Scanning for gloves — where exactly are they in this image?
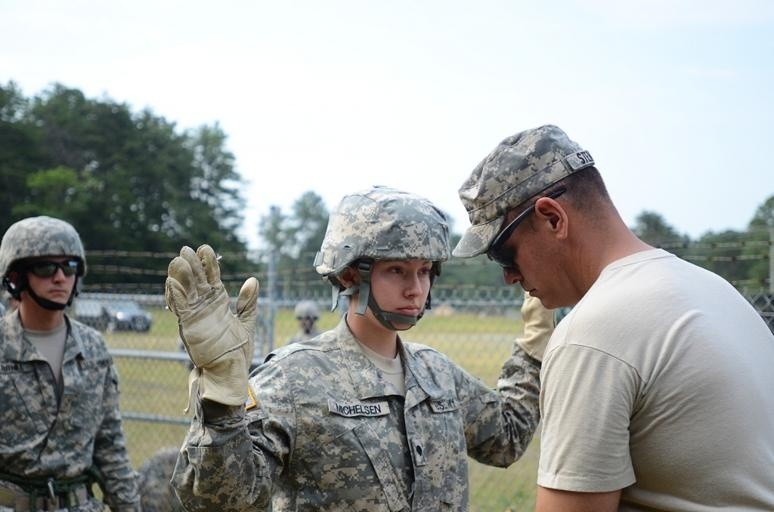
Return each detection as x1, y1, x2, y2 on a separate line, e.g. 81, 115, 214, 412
516, 291, 556, 362
165, 244, 260, 407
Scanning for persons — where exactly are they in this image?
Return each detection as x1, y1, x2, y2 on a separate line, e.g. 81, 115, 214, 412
132, 444, 186, 512
0, 215, 142, 512
164, 186, 557, 512
288, 299, 322, 343
453, 125, 774, 512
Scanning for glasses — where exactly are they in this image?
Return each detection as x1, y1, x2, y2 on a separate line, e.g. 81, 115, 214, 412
29, 260, 81, 278
486, 188, 567, 268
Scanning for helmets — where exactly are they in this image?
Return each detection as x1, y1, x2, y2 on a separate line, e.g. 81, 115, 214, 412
313, 184, 449, 276
295, 300, 320, 319
0, 216, 87, 290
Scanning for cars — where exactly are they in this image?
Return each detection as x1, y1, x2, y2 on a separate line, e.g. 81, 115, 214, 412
70, 297, 152, 332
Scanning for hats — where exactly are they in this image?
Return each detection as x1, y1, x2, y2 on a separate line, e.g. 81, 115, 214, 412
452, 124, 596, 258
